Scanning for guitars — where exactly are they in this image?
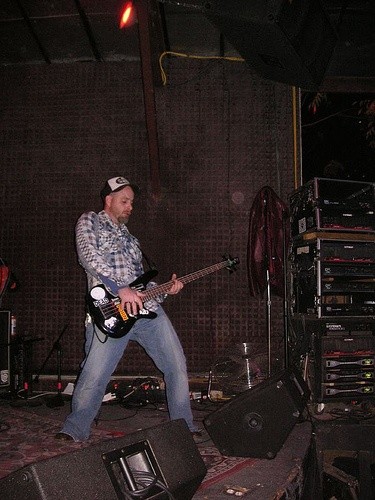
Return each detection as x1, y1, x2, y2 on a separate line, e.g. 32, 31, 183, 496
85, 254, 240, 338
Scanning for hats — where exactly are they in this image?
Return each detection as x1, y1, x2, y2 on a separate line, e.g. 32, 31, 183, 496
102, 176, 140, 199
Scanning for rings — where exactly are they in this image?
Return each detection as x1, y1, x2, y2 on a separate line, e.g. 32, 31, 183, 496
125, 302, 131, 304
132, 301, 136, 303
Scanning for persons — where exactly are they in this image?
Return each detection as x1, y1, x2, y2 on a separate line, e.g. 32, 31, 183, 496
55, 176, 204, 444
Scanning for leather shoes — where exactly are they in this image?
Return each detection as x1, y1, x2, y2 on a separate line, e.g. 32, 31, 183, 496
191, 432, 203, 438
54, 432, 74, 443
